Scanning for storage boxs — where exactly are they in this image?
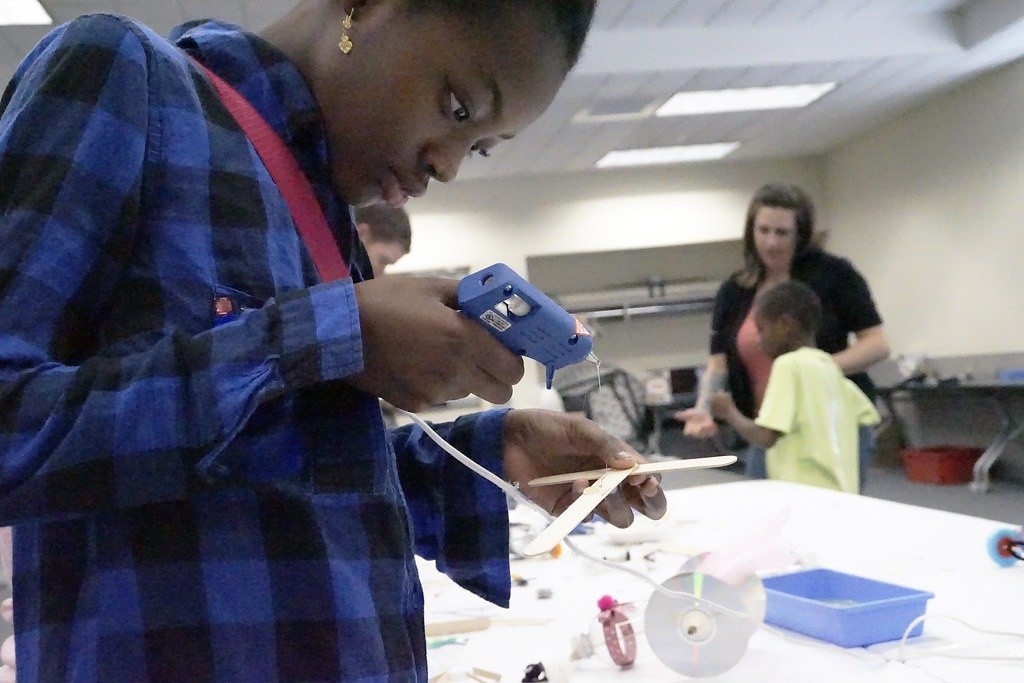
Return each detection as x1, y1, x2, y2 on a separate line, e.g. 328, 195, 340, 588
760, 567, 935, 646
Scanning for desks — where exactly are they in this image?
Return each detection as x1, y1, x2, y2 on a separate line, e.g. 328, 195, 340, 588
368, 479, 1024, 683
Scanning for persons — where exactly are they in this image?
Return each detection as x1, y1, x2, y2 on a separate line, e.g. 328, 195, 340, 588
0, 0, 667, 683
351, 201, 410, 277
675, 180, 891, 495
